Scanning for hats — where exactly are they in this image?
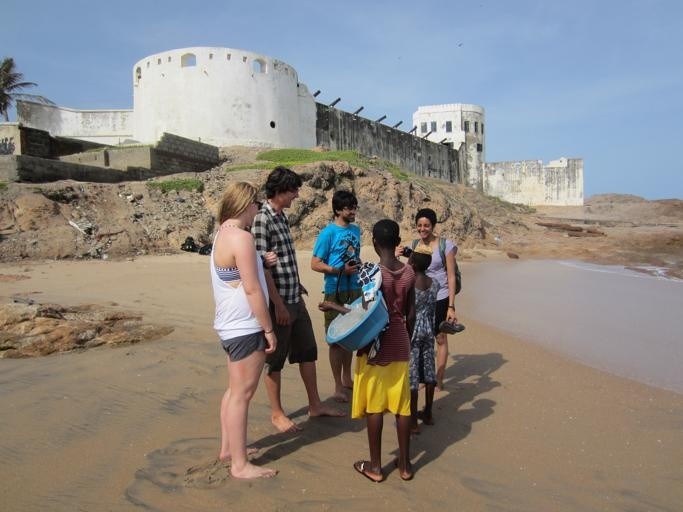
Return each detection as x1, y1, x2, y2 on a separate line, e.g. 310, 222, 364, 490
414, 245, 433, 256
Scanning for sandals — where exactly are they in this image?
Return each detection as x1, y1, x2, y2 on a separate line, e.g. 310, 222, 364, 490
439, 321, 465, 334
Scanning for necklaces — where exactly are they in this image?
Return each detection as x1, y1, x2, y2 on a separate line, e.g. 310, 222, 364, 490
217, 224, 244, 229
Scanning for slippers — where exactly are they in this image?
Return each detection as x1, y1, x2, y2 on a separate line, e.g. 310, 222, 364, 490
354, 461, 383, 482
394, 457, 413, 480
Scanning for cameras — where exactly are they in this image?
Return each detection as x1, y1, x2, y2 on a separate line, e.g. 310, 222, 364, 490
400, 246, 412, 257
348, 258, 357, 268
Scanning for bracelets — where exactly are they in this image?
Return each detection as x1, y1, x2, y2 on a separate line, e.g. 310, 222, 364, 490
447, 305, 454, 310
264, 327, 274, 336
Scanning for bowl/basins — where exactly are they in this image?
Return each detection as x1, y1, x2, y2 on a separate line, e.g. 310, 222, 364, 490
325, 289, 391, 352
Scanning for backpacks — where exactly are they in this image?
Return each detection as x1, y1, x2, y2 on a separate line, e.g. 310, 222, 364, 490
412, 238, 461, 294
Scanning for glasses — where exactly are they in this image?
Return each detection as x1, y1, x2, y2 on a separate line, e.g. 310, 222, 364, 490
344, 205, 360, 211
255, 201, 263, 210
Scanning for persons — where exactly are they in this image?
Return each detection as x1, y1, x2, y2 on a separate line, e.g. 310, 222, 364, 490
395, 207, 461, 393
209, 181, 280, 481
310, 189, 366, 405
247, 164, 348, 437
401, 244, 441, 430
316, 217, 417, 484
